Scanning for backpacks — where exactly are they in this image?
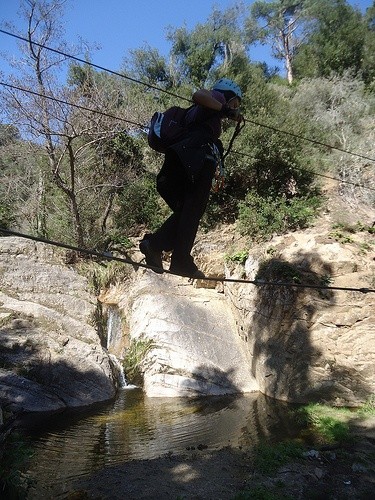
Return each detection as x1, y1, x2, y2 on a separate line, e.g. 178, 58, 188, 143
147, 106, 184, 153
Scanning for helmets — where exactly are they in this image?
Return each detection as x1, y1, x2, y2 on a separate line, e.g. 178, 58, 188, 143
212, 77, 242, 101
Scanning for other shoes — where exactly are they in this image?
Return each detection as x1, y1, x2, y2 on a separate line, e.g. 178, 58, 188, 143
169, 258, 205, 278
138, 233, 166, 274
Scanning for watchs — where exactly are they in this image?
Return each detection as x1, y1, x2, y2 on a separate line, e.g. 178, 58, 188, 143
226, 108, 232, 118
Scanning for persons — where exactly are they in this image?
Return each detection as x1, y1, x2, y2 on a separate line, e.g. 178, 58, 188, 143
139, 77, 246, 278
